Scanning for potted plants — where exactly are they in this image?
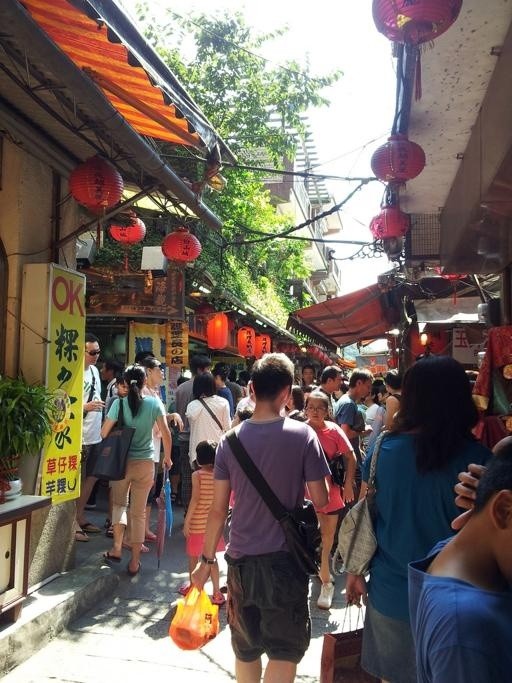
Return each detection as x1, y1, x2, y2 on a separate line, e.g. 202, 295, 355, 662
0, 367, 59, 501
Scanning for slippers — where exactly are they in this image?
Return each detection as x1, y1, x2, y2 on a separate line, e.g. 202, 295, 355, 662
75, 522, 103, 542
128, 559, 141, 577
103, 551, 121, 563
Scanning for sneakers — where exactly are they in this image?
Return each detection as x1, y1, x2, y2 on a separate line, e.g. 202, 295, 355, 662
179, 585, 190, 597
211, 592, 225, 605
318, 583, 334, 609
171, 493, 177, 503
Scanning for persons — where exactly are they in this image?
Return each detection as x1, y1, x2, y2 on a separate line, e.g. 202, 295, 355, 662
73, 333, 109, 543
98, 351, 405, 612
342, 353, 495, 683
406, 434, 512, 683
189, 351, 333, 683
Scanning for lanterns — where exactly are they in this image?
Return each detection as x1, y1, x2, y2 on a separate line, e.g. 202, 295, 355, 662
367, 205, 412, 241
206, 309, 229, 350
368, 133, 428, 193
236, 324, 256, 357
109, 211, 148, 271
278, 342, 334, 367
67, 154, 128, 250
437, 266, 468, 306
254, 333, 271, 360
160, 224, 202, 294
194, 301, 216, 326
372, 0, 463, 102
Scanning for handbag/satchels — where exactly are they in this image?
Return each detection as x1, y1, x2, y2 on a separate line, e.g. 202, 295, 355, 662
337, 496, 377, 578
279, 499, 323, 575
320, 628, 381, 683
86, 423, 136, 481
329, 454, 361, 489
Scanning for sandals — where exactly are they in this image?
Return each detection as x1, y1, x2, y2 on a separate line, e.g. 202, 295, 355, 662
104, 518, 157, 553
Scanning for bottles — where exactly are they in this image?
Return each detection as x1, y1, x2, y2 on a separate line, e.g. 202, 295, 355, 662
317, 582, 336, 610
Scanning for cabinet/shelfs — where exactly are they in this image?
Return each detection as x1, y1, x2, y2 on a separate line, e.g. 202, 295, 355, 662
0, 494, 52, 622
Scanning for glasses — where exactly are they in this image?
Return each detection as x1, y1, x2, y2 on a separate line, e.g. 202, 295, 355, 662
307, 405, 326, 412
85, 350, 101, 357
153, 364, 166, 371
